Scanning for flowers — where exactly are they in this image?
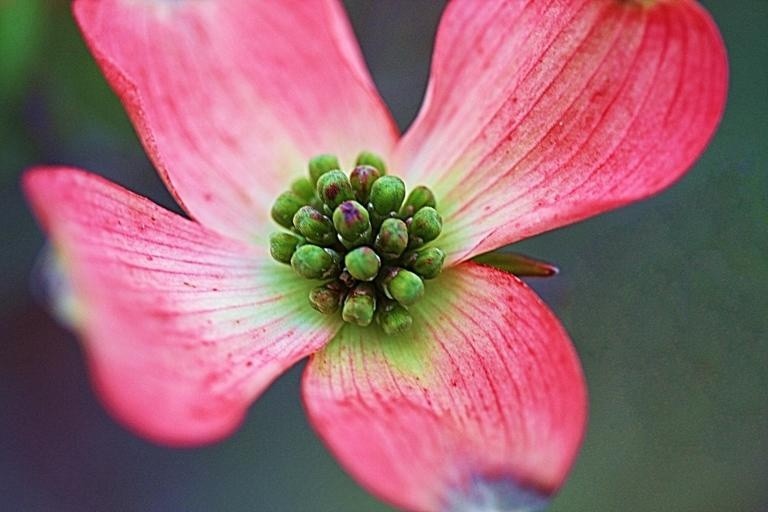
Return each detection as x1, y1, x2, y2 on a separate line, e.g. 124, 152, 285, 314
20, 0, 731, 512
